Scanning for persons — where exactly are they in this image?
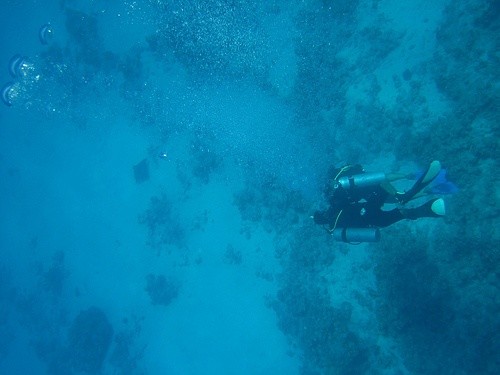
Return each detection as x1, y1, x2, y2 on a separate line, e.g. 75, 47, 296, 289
309, 158, 449, 230
